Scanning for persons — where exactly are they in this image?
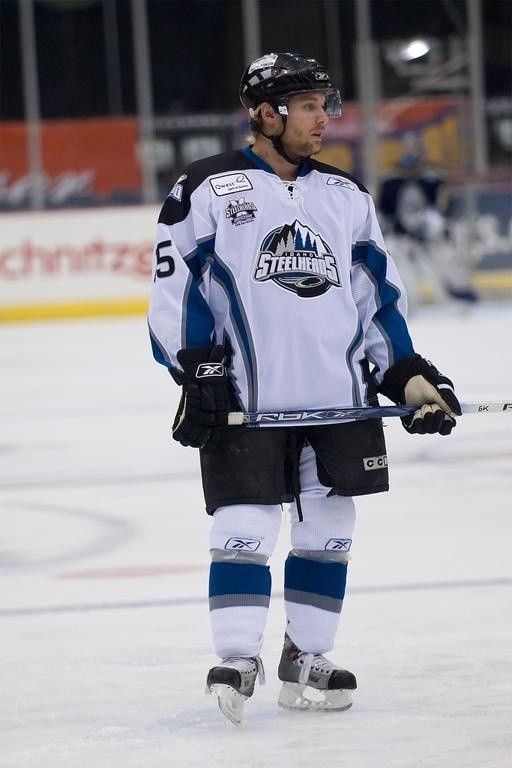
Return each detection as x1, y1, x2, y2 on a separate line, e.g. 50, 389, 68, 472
147, 49, 463, 697
376, 146, 478, 304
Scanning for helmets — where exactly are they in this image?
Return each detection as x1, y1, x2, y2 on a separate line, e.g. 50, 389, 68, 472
239, 52, 334, 115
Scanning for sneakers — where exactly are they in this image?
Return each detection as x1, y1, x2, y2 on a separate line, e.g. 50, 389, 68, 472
280, 632, 358, 691
206, 654, 266, 699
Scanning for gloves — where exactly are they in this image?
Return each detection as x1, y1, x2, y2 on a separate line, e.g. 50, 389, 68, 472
172, 344, 246, 454
376, 354, 463, 435
